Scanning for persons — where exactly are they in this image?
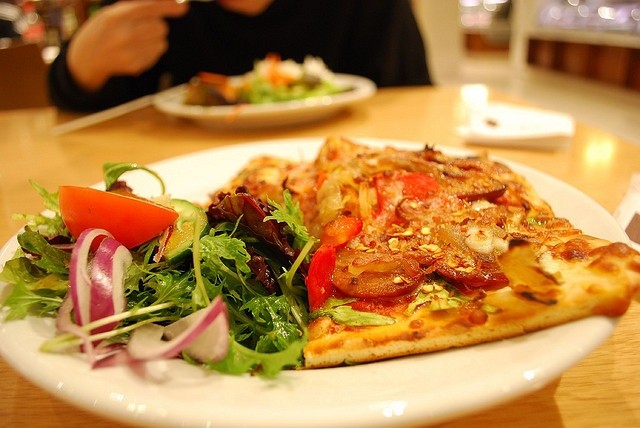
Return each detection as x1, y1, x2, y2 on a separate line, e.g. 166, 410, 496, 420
47, 0, 432, 114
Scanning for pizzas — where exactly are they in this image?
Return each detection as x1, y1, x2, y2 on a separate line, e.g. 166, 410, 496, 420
215, 150, 316, 235
300, 131, 637, 371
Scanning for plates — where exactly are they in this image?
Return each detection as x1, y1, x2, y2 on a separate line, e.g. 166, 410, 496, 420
0, 133, 640, 427
150, 68, 379, 132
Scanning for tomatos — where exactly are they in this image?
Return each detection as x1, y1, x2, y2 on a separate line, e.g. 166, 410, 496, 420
56, 184, 180, 258
